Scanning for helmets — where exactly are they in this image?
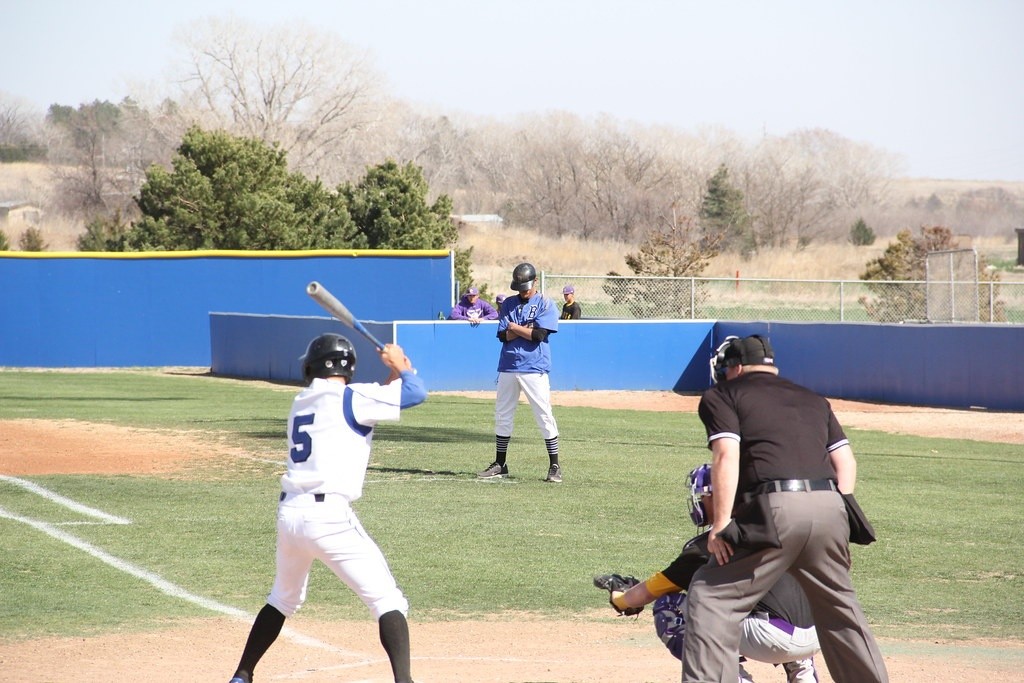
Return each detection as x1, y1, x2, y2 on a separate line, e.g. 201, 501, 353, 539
510, 263, 536, 290
299, 334, 357, 383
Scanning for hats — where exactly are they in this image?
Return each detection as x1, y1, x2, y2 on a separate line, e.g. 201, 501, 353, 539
496, 295, 506, 303
717, 334, 775, 367
561, 286, 574, 294
466, 288, 477, 296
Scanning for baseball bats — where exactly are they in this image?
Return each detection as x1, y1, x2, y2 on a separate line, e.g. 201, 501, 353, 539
305, 279, 418, 376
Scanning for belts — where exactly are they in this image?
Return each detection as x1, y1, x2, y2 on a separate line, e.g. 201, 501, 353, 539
769, 618, 795, 635
767, 479, 839, 492
280, 492, 325, 502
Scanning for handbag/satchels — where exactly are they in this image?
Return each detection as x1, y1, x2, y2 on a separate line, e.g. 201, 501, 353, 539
716, 492, 782, 549
842, 494, 876, 544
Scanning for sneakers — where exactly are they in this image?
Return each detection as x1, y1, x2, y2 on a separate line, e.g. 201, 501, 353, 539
478, 462, 508, 479
547, 464, 562, 483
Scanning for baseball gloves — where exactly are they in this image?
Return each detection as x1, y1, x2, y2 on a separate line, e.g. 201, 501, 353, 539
593, 573, 644, 615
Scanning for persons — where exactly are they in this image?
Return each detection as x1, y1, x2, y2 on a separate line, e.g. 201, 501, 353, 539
561, 286, 581, 319
496, 294, 506, 310
681, 331, 890, 683
228, 334, 428, 683
479, 264, 562, 482
451, 287, 498, 323
611, 464, 821, 683
